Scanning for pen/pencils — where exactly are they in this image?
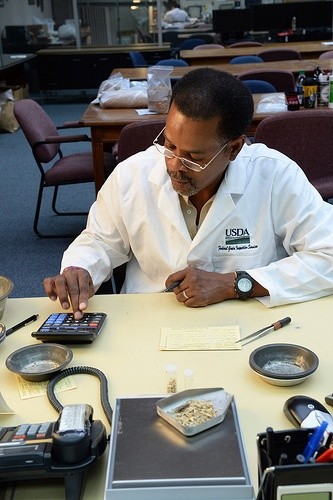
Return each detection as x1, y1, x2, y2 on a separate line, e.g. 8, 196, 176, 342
266, 421, 333, 465
6, 314, 39, 336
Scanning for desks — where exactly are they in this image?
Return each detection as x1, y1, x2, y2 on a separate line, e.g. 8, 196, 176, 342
110, 59, 333, 89
80, 92, 333, 201
177, 40, 333, 65
0, 53, 38, 85
0, 290, 333, 500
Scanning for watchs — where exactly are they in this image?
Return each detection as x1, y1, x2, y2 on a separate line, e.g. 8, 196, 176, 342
234, 271, 254, 300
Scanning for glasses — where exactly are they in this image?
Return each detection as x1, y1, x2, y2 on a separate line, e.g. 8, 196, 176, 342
152, 126, 227, 172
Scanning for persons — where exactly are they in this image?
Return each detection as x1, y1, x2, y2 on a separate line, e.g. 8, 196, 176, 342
42, 68, 333, 319
162, 2, 192, 23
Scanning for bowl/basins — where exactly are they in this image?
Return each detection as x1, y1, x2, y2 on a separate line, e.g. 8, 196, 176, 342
248, 343, 319, 386
5, 343, 74, 382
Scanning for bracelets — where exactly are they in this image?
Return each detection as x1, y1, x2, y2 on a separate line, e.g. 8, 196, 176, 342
63, 267, 81, 271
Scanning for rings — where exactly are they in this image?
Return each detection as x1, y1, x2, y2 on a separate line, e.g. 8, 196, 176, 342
183, 290, 189, 299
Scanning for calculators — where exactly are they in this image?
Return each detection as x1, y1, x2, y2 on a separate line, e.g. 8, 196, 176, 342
32, 313, 107, 344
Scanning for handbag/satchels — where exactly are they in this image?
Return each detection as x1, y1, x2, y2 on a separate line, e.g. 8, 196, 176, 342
0, 100, 20, 133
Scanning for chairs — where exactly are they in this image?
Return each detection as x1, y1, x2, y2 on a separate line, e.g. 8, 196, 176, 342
14, 35, 333, 237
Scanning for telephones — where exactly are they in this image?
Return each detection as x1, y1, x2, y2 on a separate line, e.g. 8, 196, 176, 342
0, 404, 107, 500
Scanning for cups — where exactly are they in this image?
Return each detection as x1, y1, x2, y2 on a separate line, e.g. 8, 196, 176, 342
284, 91, 301, 111
302, 78, 318, 109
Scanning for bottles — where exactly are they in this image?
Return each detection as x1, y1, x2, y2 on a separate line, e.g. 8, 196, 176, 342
314, 66, 333, 108
295, 69, 306, 104
292, 17, 296, 30
